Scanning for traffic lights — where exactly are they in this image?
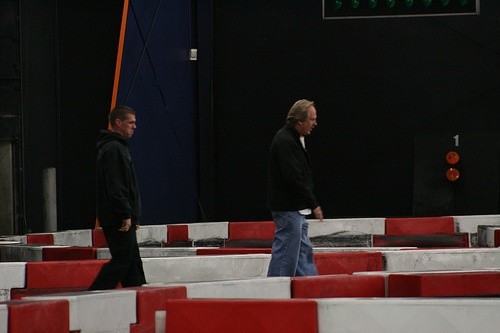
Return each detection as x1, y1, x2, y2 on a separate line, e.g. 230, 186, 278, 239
429, 145, 467, 183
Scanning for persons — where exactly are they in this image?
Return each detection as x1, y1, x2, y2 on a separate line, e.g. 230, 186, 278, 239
266, 99, 324, 278
88, 103, 147, 292
